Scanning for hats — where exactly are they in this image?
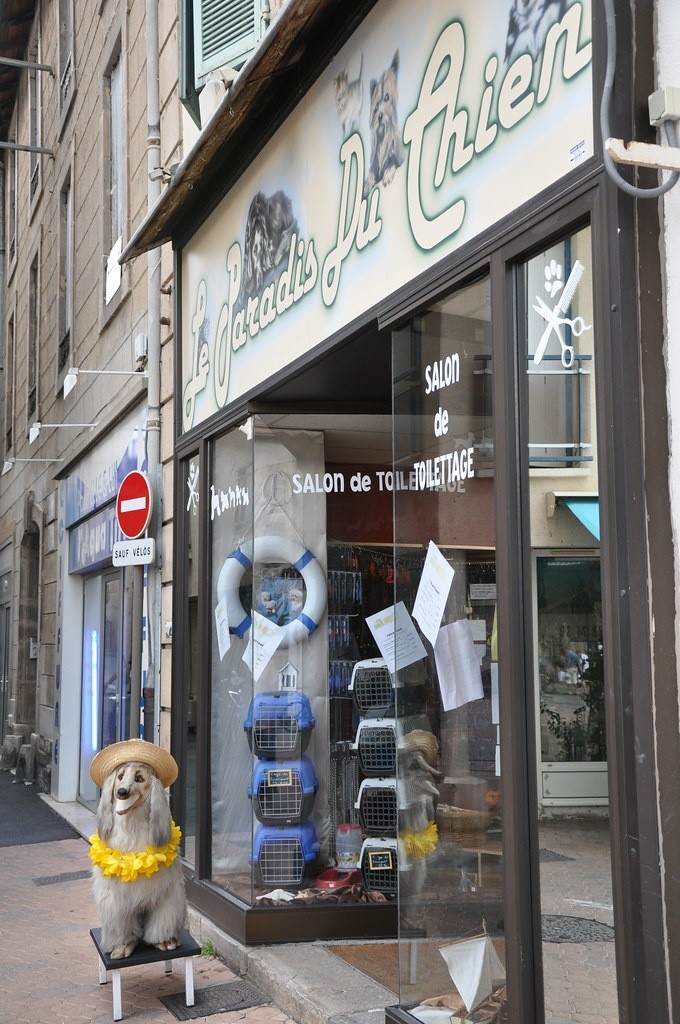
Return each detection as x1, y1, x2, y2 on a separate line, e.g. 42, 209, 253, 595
90, 739, 178, 788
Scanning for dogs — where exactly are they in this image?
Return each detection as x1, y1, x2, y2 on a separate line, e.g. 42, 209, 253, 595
87, 760, 189, 960
398, 751, 446, 930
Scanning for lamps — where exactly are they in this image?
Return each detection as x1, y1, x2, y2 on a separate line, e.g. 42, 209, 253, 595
63, 367, 147, 401
1, 457, 63, 478
29, 422, 96, 447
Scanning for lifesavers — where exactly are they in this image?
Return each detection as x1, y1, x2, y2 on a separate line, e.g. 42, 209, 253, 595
215, 534, 329, 651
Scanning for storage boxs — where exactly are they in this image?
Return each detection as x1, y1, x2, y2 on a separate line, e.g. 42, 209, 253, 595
243, 690, 322, 889
348, 659, 434, 898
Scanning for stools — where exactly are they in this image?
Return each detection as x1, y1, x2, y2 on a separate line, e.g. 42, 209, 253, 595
90, 925, 201, 1022
